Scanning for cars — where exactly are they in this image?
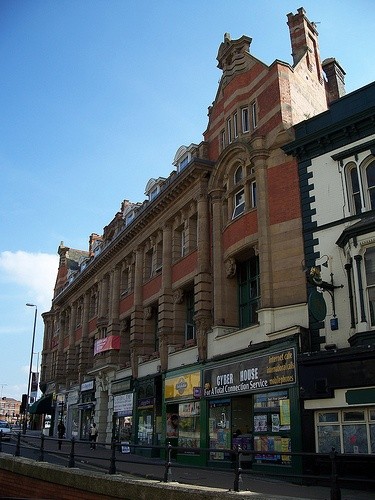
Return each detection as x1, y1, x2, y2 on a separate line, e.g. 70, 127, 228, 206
0, 421, 13, 440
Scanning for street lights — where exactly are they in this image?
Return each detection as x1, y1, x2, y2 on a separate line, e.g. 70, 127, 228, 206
23, 303, 37, 436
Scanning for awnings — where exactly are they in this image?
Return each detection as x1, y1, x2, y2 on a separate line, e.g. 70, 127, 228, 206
29, 392, 55, 415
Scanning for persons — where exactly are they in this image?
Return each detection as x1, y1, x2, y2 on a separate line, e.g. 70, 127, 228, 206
58, 420, 66, 444
89, 422, 98, 450
